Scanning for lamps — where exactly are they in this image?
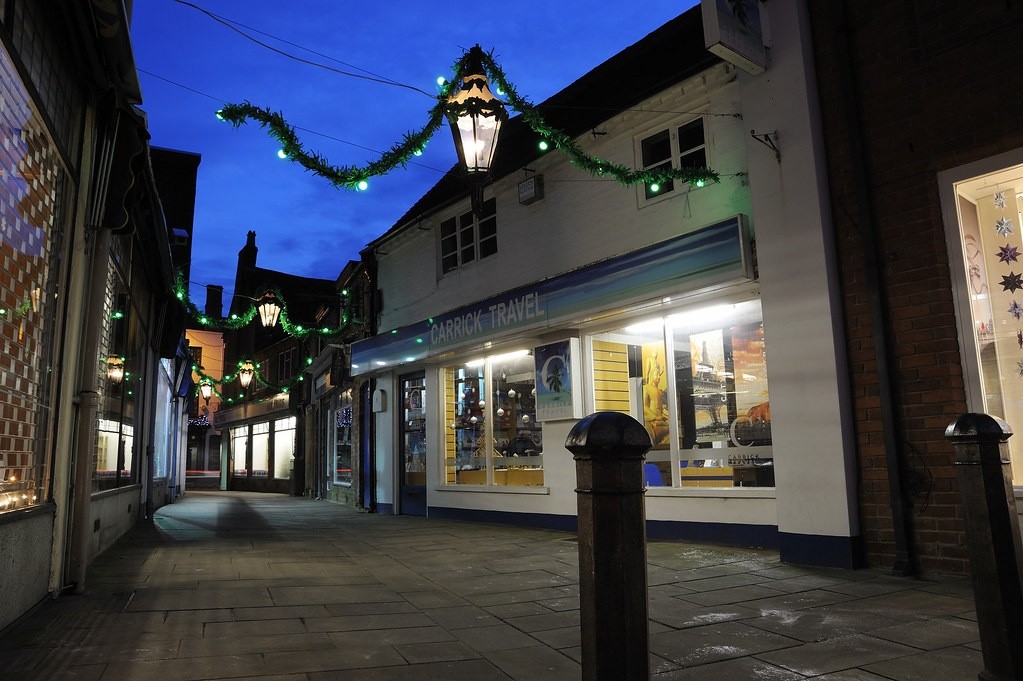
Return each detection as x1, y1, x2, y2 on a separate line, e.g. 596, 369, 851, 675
200, 381, 212, 405
238, 358, 255, 389
255, 284, 283, 336
443, 43, 498, 218
